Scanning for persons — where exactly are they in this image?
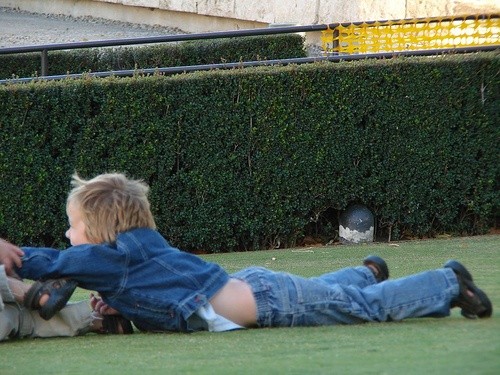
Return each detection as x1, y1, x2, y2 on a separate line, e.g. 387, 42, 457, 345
0, 236, 135, 341
0, 172, 493, 334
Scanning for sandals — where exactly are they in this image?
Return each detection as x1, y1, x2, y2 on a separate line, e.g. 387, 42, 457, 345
363, 254, 390, 282
444, 260, 492, 319
100, 314, 133, 334
32, 278, 79, 320
